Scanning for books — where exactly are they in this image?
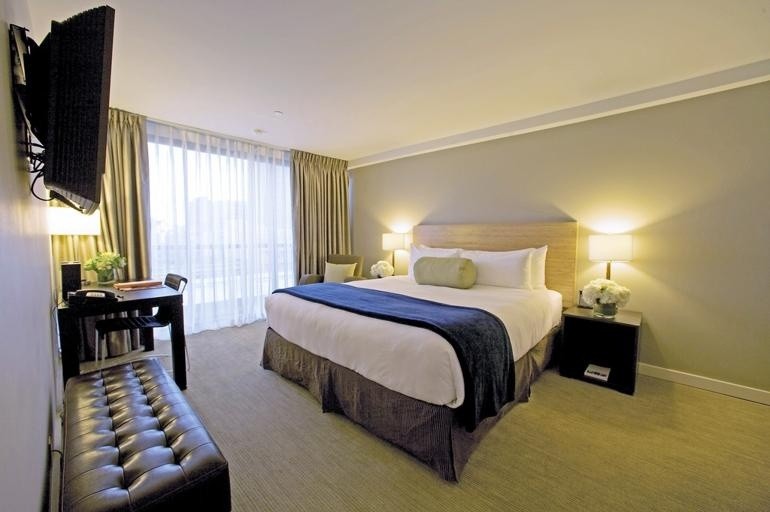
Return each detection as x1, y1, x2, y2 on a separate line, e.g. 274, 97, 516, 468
114, 280, 164, 292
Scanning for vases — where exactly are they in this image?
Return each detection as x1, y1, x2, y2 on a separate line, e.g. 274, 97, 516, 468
97, 270, 115, 285
593, 302, 616, 318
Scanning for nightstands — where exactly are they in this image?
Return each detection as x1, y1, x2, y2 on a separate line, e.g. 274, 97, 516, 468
560, 305, 643, 396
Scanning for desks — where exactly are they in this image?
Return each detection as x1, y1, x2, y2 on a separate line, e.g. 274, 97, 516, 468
58, 280, 186, 390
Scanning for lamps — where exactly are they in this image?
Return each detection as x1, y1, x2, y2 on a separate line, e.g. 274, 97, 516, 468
382, 233, 406, 268
48, 207, 101, 262
588, 235, 633, 280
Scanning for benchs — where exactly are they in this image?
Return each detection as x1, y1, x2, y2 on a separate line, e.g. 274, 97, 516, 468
62, 357, 231, 512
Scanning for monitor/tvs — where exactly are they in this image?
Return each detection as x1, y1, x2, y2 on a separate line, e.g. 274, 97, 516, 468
21, 5, 116, 217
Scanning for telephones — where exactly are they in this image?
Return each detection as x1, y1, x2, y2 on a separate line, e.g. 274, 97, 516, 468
67, 289, 117, 305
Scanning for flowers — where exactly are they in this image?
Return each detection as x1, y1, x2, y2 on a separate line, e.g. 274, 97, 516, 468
83, 251, 126, 270
583, 278, 630, 309
370, 261, 395, 278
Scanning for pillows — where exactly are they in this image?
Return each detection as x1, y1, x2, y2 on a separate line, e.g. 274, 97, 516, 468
324, 260, 357, 284
420, 245, 462, 255
530, 245, 548, 291
459, 248, 535, 288
408, 244, 458, 286
414, 257, 477, 289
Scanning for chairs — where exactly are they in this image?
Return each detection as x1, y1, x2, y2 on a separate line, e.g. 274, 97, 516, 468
95, 274, 188, 371
298, 254, 367, 284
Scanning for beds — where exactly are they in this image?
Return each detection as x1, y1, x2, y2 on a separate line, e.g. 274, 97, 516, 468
265, 223, 579, 470
